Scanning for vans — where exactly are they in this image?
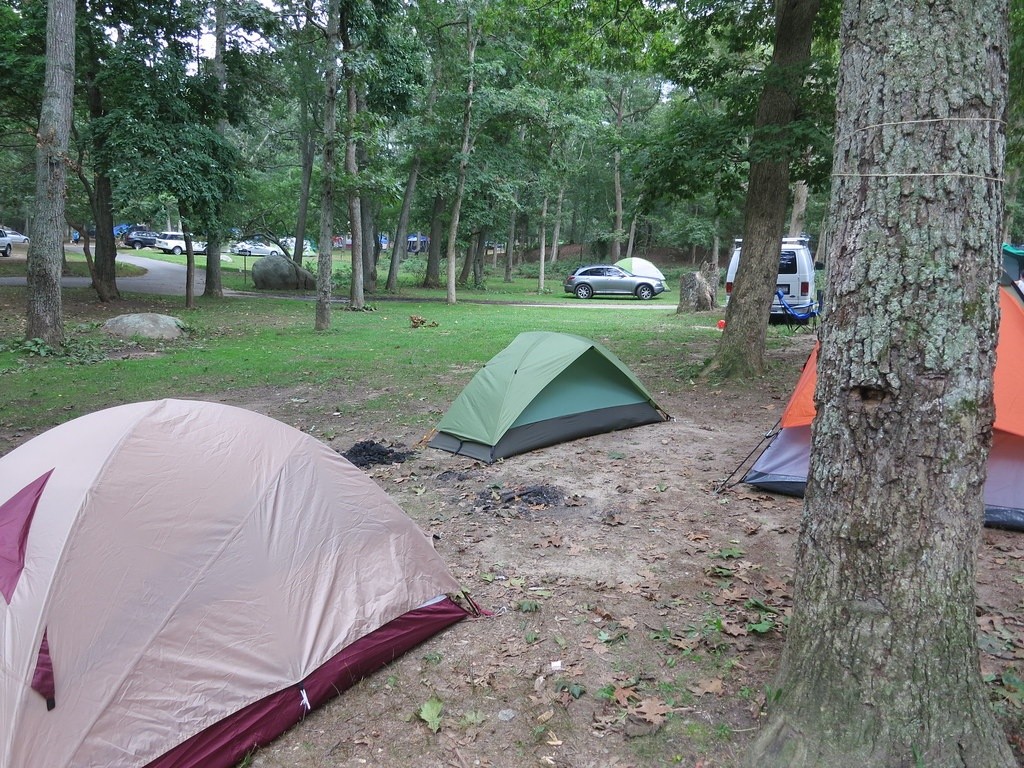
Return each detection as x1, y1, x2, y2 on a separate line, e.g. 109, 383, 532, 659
726, 238, 812, 317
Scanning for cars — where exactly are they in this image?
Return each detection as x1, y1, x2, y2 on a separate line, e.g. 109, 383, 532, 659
5, 231, 30, 243
231, 242, 279, 256
0, 229, 12, 256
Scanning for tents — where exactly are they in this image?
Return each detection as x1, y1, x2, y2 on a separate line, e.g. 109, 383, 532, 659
425, 328, 672, 465
613, 257, 671, 292
0, 396, 481, 768
742, 255, 1024, 533
373, 232, 432, 253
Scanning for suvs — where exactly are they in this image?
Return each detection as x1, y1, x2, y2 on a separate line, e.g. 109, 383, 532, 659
124, 231, 160, 248
565, 265, 663, 300
155, 232, 207, 255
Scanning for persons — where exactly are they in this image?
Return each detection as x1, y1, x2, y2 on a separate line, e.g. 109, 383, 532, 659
73, 230, 81, 246
1012, 270, 1024, 294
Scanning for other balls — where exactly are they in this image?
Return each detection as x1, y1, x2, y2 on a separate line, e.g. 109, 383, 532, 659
717, 319, 725, 329
